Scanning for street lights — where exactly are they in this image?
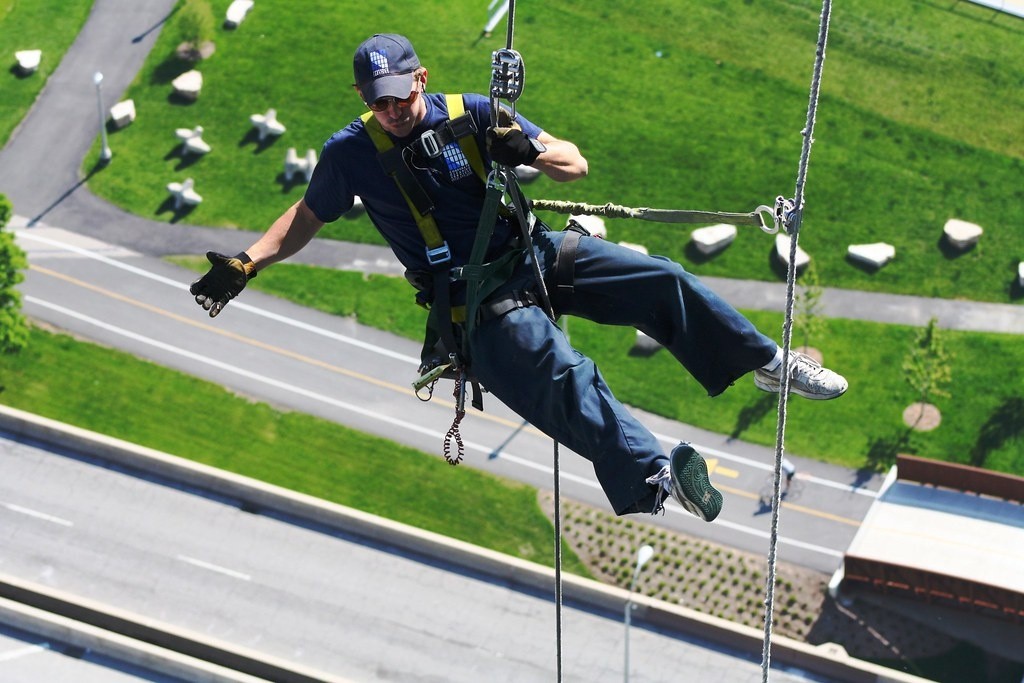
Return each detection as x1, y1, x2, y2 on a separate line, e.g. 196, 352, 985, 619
92, 71, 113, 163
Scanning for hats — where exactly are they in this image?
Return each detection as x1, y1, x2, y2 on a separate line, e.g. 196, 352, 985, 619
353, 33, 420, 106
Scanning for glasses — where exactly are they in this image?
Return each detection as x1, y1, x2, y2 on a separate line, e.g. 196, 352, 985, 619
361, 74, 421, 112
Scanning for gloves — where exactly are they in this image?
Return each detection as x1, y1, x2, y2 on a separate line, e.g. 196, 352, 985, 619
486, 106, 546, 167
190, 250, 258, 318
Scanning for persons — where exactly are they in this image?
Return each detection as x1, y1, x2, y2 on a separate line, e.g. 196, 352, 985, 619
189, 33, 849, 523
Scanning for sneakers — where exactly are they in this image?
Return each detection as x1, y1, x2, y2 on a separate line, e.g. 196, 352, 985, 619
754, 350, 848, 400
646, 443, 723, 523
